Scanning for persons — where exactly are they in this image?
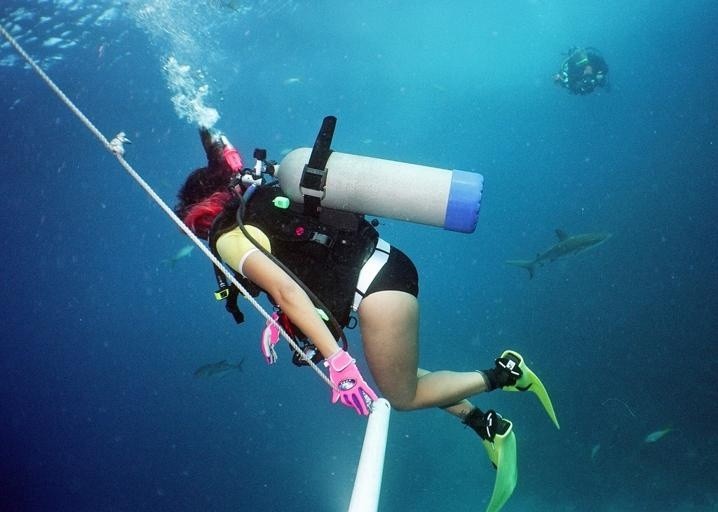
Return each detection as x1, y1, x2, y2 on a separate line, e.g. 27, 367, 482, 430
552, 45, 609, 95
172, 167, 562, 512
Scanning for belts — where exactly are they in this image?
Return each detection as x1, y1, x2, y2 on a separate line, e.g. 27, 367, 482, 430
352, 236, 391, 313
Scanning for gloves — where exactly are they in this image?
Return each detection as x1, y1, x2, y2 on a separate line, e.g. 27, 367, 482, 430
260, 314, 282, 364
324, 348, 378, 417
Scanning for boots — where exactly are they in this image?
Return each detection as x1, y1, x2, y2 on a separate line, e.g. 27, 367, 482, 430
474, 348, 562, 430
463, 408, 518, 512
